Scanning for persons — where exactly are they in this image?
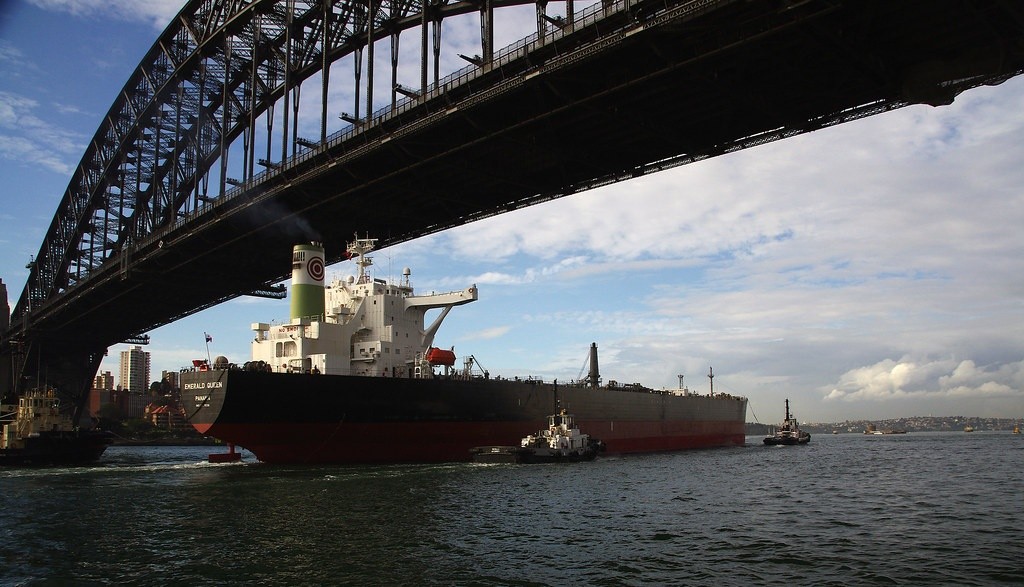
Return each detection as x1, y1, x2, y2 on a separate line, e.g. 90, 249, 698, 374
286, 365, 293, 373
312, 365, 320, 373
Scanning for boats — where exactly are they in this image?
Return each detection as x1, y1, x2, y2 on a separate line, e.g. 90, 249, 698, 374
179, 231, 749, 466
763, 398, 811, 445
0, 387, 115, 469
964, 425, 973, 432
520, 378, 602, 463
832, 431, 837, 434
1013, 427, 1021, 434
468, 445, 536, 464
863, 424, 908, 434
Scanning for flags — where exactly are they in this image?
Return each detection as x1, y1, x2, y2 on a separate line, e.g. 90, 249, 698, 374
205, 334, 212, 343
